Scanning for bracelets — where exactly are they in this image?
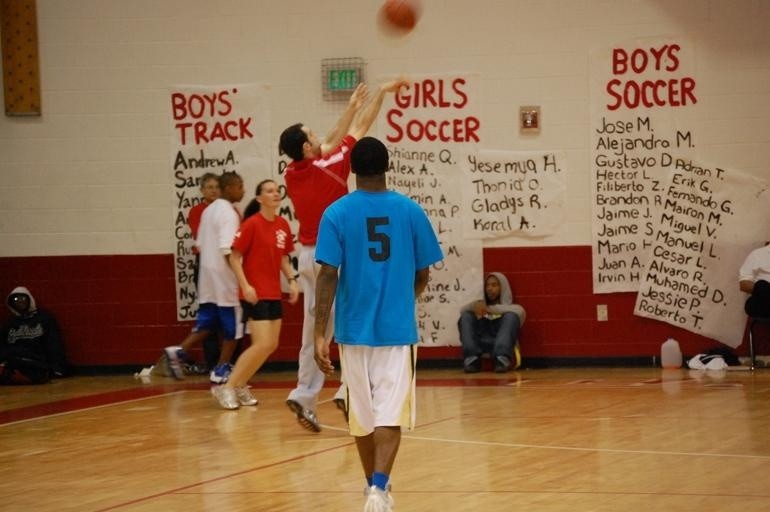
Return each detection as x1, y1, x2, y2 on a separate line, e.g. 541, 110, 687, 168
287, 278, 295, 283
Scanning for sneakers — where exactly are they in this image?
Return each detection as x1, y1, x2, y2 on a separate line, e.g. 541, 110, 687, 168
165, 346, 259, 410
495, 363, 505, 373
364, 485, 392, 512
334, 388, 348, 420
286, 398, 321, 432
464, 362, 480, 373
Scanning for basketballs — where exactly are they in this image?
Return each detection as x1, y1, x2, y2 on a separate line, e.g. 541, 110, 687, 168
376, 1, 422, 36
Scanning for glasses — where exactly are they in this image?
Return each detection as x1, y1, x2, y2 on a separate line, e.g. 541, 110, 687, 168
13, 297, 27, 302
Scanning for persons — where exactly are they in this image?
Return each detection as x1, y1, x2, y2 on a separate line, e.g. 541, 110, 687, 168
161, 173, 253, 382
0, 280, 64, 386
459, 271, 526, 374
189, 174, 246, 366
211, 180, 300, 410
738, 241, 770, 319
279, 79, 410, 431
310, 138, 443, 511
